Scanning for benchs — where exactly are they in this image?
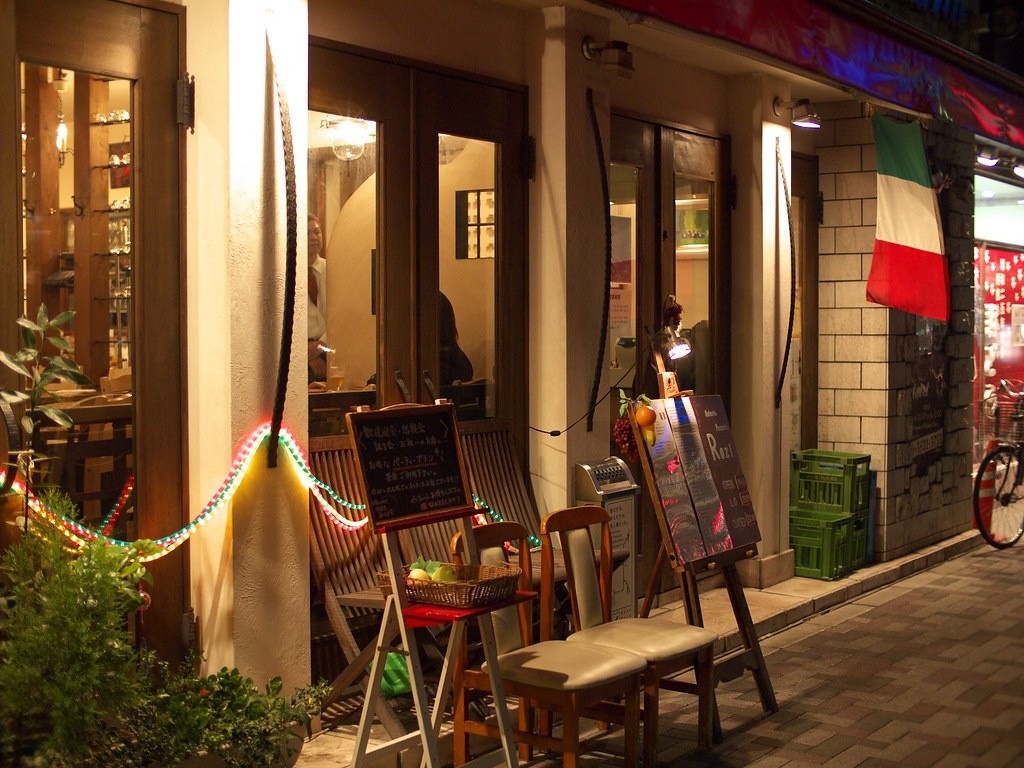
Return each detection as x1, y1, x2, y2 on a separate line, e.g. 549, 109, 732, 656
309, 419, 631, 748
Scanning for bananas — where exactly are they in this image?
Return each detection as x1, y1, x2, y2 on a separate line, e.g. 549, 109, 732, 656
640, 400, 656, 446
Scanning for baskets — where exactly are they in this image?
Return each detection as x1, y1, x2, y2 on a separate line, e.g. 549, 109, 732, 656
979, 400, 1024, 444
379, 562, 523, 608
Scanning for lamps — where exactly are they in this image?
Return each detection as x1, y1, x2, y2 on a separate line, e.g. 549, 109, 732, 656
332, 116, 365, 161
1013, 159, 1024, 180
772, 95, 826, 133
660, 316, 699, 364
50, 113, 71, 168
976, 145, 1001, 168
584, 34, 639, 79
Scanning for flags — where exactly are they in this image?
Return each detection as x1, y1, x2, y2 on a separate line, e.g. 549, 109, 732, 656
867, 113, 950, 322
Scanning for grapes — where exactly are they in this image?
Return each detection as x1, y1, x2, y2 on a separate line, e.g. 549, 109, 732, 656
613, 417, 637, 455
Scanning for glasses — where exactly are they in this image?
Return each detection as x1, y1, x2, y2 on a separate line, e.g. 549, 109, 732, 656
96, 108, 134, 299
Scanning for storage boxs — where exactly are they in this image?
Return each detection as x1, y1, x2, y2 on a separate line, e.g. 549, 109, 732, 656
790, 448, 881, 581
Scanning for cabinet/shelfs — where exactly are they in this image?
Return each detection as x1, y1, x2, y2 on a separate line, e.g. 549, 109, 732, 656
87, 118, 130, 346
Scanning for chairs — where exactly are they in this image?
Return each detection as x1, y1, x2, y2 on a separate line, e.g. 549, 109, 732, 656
536, 506, 721, 768
445, 521, 649, 768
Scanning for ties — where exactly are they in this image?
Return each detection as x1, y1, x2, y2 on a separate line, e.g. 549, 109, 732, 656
308, 266, 318, 305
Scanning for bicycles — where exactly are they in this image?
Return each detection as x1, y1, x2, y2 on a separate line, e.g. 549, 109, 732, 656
972, 378, 1024, 550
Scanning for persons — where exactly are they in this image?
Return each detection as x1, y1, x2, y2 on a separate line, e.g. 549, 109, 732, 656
307, 214, 327, 384
438, 291, 473, 385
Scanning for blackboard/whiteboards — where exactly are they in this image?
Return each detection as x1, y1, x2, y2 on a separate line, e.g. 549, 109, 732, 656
346, 403, 474, 532
627, 395, 762, 572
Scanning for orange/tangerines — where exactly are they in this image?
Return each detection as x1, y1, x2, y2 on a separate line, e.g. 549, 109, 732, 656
407, 569, 430, 587
635, 405, 656, 427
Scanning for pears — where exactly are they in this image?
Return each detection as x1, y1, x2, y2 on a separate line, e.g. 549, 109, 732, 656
431, 566, 456, 589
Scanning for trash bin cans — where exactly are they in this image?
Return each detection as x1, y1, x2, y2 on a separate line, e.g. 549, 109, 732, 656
574, 456, 641, 618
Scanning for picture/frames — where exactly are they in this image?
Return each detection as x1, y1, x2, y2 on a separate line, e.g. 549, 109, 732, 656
675, 200, 709, 259
